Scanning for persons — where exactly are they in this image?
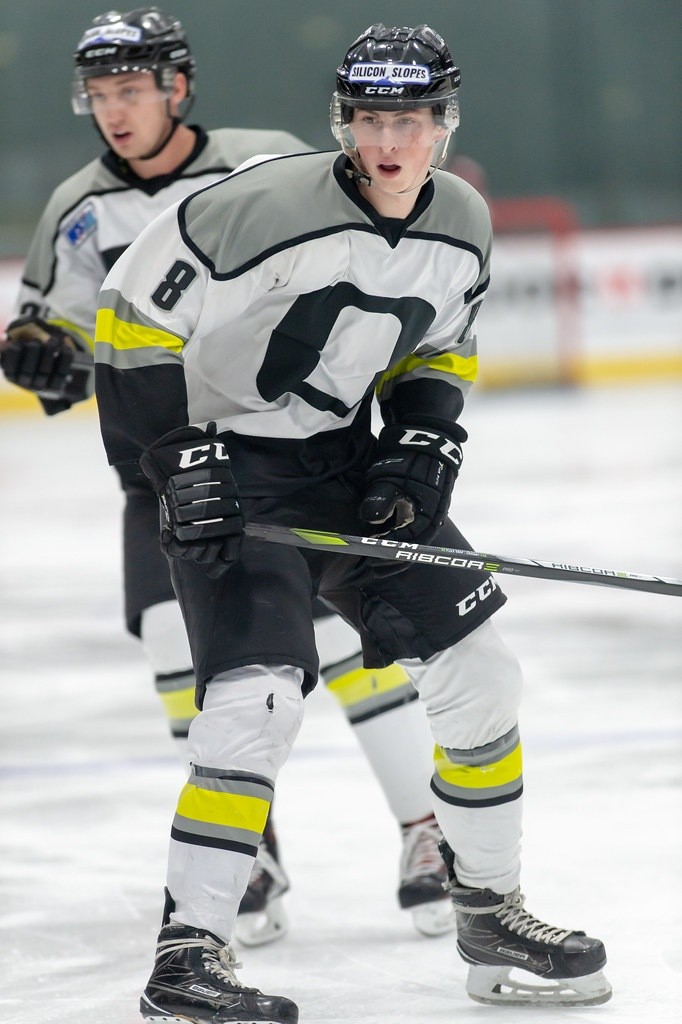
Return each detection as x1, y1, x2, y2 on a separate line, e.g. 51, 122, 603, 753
0, 9, 456, 947
96, 22, 614, 1023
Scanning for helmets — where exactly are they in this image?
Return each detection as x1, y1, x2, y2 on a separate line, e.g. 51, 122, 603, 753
337, 22, 461, 130
74, 6, 195, 98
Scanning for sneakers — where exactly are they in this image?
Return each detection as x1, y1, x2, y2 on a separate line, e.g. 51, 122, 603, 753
398, 811, 459, 938
235, 815, 290, 946
437, 837, 612, 1009
140, 886, 299, 1024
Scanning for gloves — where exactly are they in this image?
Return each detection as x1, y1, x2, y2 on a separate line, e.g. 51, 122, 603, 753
0, 317, 89, 401
360, 413, 468, 578
139, 421, 246, 579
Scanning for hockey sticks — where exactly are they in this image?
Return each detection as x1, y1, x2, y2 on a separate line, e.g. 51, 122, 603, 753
0, 340, 94, 371
242, 521, 682, 599
